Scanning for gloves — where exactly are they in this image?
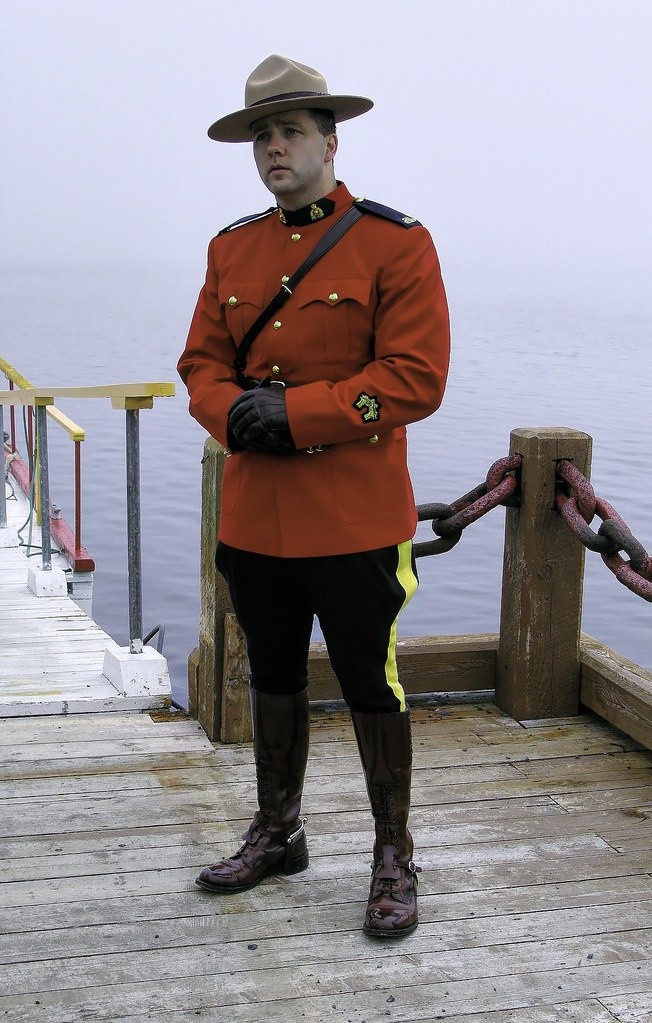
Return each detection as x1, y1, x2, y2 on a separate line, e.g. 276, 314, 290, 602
229, 387, 296, 456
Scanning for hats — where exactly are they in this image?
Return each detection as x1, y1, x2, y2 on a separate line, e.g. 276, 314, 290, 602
208, 54, 374, 144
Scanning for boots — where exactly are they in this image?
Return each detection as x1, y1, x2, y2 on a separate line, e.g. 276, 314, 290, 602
196, 685, 309, 894
350, 702, 423, 937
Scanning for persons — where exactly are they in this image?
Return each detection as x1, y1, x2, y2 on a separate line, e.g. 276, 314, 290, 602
176, 54, 451, 935
